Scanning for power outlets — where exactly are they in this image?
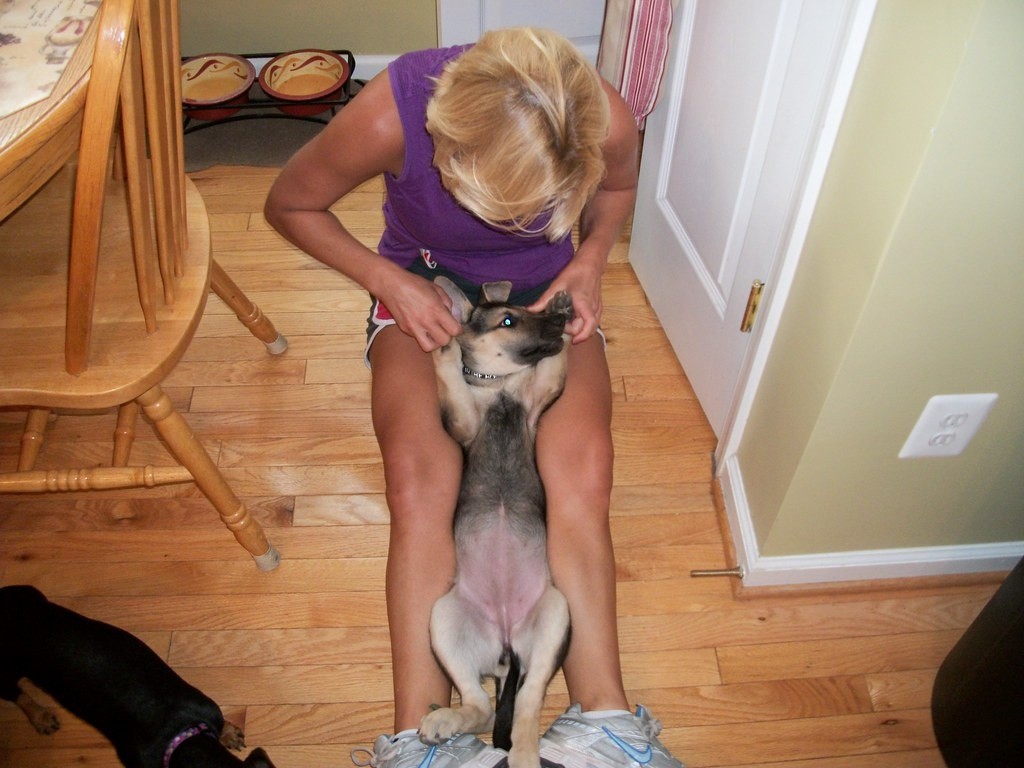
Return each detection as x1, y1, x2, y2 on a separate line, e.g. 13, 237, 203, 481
898, 392, 999, 459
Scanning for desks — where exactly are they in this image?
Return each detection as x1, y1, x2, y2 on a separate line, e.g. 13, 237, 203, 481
0, 0, 115, 224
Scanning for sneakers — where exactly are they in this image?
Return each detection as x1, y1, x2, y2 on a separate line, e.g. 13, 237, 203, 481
350, 729, 508, 768
539, 703, 689, 768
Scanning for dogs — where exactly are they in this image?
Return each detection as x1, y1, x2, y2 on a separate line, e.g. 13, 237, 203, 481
416, 275, 573, 768
0, 584, 276, 768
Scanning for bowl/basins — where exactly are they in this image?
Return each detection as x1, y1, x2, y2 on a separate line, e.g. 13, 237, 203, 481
258, 48, 350, 117
178, 52, 256, 122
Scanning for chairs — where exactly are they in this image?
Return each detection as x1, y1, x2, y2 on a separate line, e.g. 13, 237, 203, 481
0, 0, 289, 575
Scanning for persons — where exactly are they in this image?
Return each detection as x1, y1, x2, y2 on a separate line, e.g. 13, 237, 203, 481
262, 27, 688, 768
